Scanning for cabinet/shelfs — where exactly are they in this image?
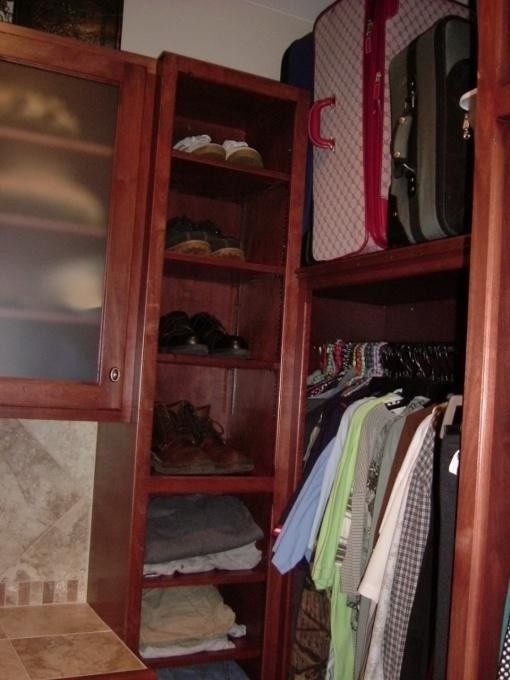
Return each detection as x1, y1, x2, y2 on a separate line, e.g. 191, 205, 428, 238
280, 1, 510, 680
0, 23, 161, 430
88, 51, 303, 662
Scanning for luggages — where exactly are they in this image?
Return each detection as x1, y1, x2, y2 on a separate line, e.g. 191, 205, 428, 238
171, 131, 227, 161
387, 11, 475, 245
306, 0, 471, 263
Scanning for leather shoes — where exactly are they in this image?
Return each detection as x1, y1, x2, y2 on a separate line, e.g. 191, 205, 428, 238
189, 310, 252, 359
156, 307, 213, 359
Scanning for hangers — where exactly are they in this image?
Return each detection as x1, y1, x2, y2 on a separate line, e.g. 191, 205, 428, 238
305, 336, 463, 404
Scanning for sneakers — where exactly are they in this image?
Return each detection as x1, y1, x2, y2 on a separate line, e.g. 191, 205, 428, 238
199, 217, 246, 262
164, 211, 213, 258
147, 400, 224, 476
190, 402, 257, 475
221, 138, 264, 168
